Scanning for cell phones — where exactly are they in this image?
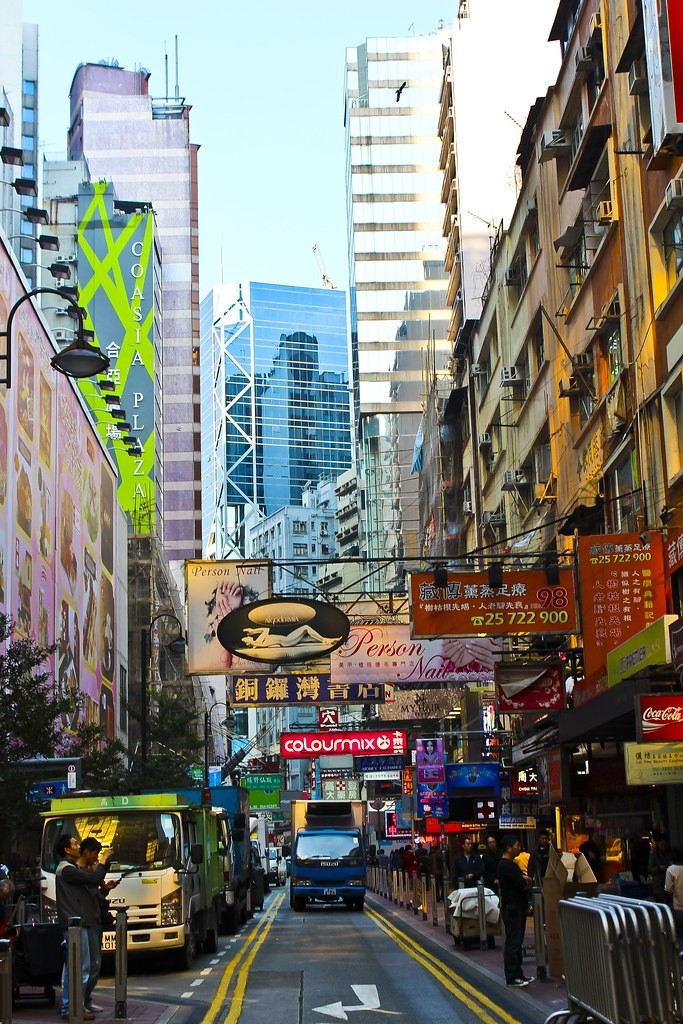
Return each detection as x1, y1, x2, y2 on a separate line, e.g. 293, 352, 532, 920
112, 878, 122, 887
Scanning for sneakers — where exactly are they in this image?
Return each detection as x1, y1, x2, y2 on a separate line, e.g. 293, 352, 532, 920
507, 976, 535, 987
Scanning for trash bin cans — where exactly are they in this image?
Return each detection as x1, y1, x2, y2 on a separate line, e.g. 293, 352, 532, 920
618, 879, 653, 901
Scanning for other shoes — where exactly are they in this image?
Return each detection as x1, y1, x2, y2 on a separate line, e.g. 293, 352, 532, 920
82, 1012, 95, 1020
90, 1004, 104, 1012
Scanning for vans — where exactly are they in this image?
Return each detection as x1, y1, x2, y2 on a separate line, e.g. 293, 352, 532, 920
269, 847, 287, 886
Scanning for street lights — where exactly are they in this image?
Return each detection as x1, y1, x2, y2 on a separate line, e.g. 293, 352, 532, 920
141, 614, 186, 769
204, 702, 237, 787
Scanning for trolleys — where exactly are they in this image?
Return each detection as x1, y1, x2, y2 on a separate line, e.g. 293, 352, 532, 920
450, 895, 501, 949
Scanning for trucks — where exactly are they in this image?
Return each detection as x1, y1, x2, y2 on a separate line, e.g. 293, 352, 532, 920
282, 800, 377, 911
142, 786, 250, 934
250, 816, 269, 892
23, 792, 246, 971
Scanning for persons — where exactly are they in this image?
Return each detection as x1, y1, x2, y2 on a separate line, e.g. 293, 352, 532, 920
366, 829, 549, 928
438, 638, 504, 672
420, 740, 441, 779
210, 581, 245, 667
241, 625, 342, 647
497, 835, 535, 987
648, 836, 683, 958
26, 833, 120, 1020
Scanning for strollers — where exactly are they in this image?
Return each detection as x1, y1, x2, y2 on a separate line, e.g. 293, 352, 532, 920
2, 922, 69, 1004
